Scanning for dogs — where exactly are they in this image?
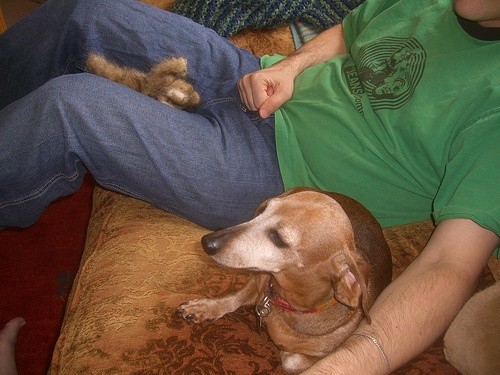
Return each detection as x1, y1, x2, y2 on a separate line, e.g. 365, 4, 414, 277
175, 186, 393, 375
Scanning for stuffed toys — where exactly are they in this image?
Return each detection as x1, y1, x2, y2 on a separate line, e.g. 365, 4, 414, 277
84, 49, 201, 109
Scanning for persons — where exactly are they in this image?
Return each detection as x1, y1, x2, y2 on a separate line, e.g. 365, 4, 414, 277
0, 0, 500, 375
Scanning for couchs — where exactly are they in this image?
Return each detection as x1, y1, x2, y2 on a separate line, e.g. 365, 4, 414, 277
47, 0, 500, 375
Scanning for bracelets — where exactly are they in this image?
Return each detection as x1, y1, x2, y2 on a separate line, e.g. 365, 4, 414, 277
348, 332, 393, 374
